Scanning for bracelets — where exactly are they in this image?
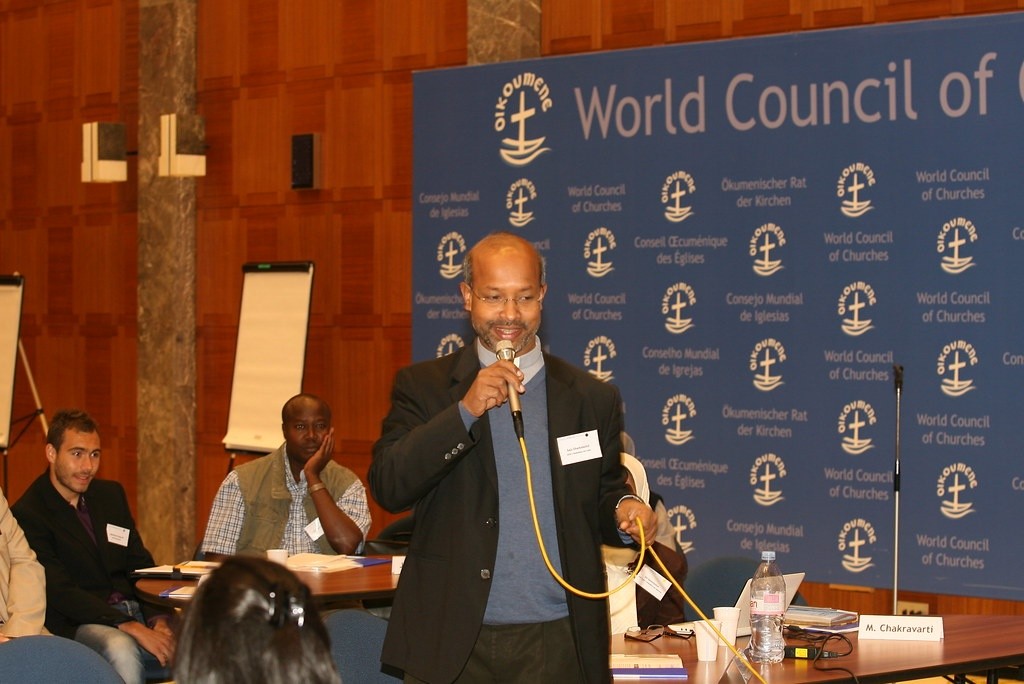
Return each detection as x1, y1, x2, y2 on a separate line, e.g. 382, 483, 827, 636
308, 483, 325, 494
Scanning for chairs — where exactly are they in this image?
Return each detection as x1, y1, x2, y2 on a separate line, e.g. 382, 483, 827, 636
360, 520, 430, 623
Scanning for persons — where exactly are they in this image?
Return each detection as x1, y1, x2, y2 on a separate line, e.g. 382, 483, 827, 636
362, 230, 677, 684
203, 392, 373, 559
0, 409, 345, 684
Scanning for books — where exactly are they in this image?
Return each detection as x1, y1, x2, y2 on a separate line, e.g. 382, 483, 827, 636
783, 604, 859, 634
159, 587, 196, 598
611, 654, 688, 678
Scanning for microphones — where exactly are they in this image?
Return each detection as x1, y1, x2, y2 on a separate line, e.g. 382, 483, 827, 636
495, 339, 524, 440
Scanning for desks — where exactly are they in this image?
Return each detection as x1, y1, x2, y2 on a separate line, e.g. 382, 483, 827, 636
134, 553, 413, 611
597, 615, 1024, 684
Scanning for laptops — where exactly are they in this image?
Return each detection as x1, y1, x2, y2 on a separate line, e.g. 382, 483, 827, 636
667, 573, 806, 637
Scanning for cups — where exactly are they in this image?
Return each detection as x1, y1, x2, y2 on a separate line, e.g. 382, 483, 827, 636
266, 549, 288, 568
712, 606, 741, 646
693, 620, 722, 662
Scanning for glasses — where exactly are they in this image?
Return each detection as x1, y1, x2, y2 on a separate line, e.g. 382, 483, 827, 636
624, 625, 694, 642
470, 284, 544, 311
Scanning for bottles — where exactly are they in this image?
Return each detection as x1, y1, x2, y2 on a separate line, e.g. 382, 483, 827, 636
749, 551, 786, 664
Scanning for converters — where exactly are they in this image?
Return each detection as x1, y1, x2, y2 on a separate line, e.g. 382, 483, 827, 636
784, 645, 822, 659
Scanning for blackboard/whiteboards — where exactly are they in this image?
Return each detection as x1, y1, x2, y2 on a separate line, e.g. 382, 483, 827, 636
0, 274, 25, 449
222, 259, 315, 456
